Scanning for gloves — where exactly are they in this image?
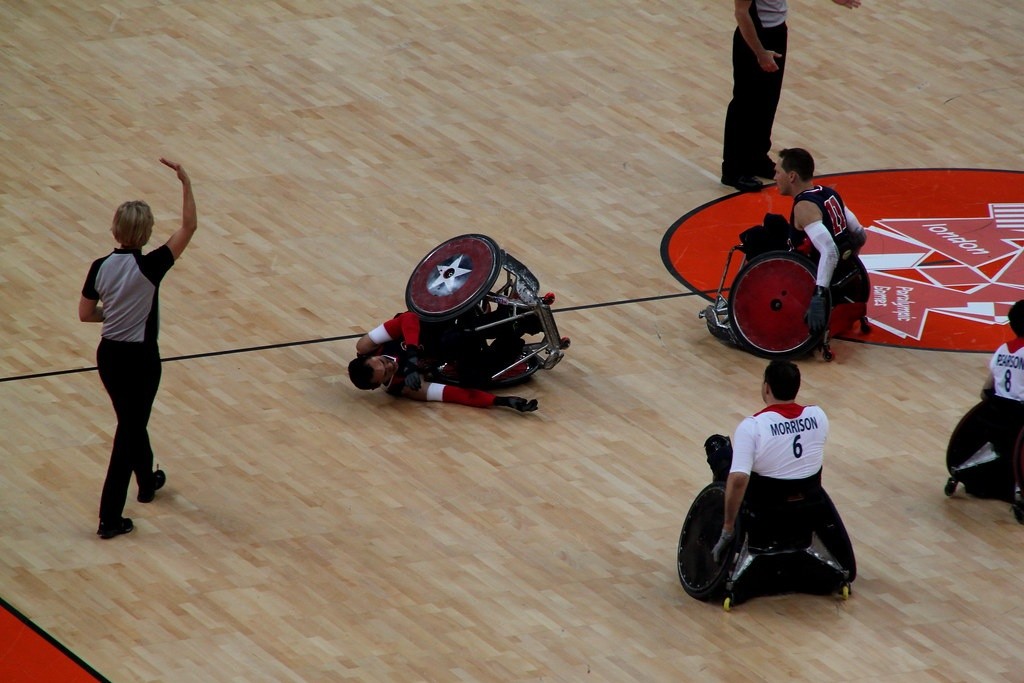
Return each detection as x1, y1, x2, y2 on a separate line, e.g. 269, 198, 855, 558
711, 530, 733, 563
404, 345, 421, 392
494, 395, 539, 412
804, 285, 829, 331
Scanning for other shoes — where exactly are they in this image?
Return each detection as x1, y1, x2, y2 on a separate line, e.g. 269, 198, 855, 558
137, 470, 166, 503
97, 518, 133, 537
756, 161, 776, 180
721, 175, 764, 192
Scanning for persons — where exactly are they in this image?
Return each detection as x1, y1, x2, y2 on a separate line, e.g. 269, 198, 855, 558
347, 294, 543, 413
712, 355, 829, 562
980, 300, 1024, 405
721, 1, 863, 193
78, 158, 198, 541
731, 147, 867, 337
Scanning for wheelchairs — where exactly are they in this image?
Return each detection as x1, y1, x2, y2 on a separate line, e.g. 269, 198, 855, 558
698, 244, 873, 363
676, 433, 856, 611
943, 400, 1024, 524
405, 233, 571, 392
